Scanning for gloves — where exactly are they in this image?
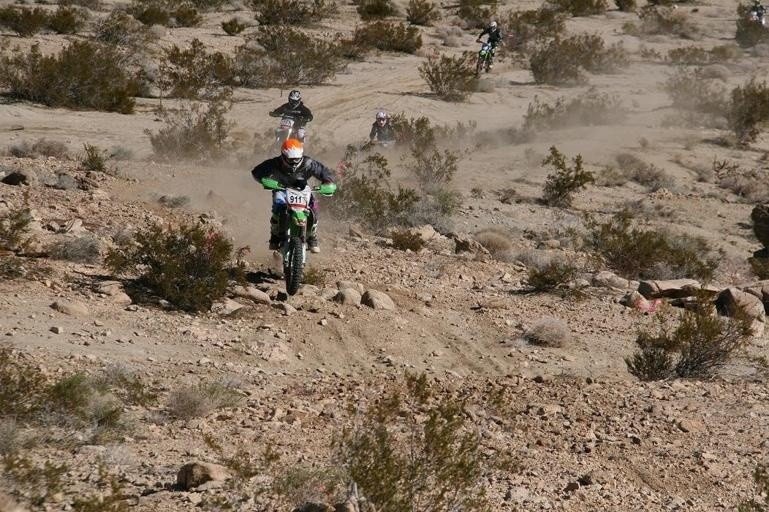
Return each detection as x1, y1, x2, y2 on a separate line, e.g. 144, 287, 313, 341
262, 177, 285, 191
320, 182, 337, 197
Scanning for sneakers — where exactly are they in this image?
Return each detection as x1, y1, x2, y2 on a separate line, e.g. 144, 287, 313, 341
269, 235, 279, 250
307, 235, 320, 253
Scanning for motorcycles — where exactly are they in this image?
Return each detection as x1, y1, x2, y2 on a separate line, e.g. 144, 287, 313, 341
746, 0, 769, 25
261, 178, 337, 295
476, 40, 497, 75
271, 114, 305, 151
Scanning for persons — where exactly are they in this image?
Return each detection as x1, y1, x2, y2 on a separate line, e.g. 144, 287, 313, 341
749, 0, 768, 23
251, 139, 334, 253
370, 112, 397, 141
269, 90, 313, 143
476, 21, 503, 69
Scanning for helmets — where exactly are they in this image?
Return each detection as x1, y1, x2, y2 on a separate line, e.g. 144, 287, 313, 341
376, 112, 387, 128
489, 21, 498, 33
288, 90, 301, 110
281, 139, 304, 172
